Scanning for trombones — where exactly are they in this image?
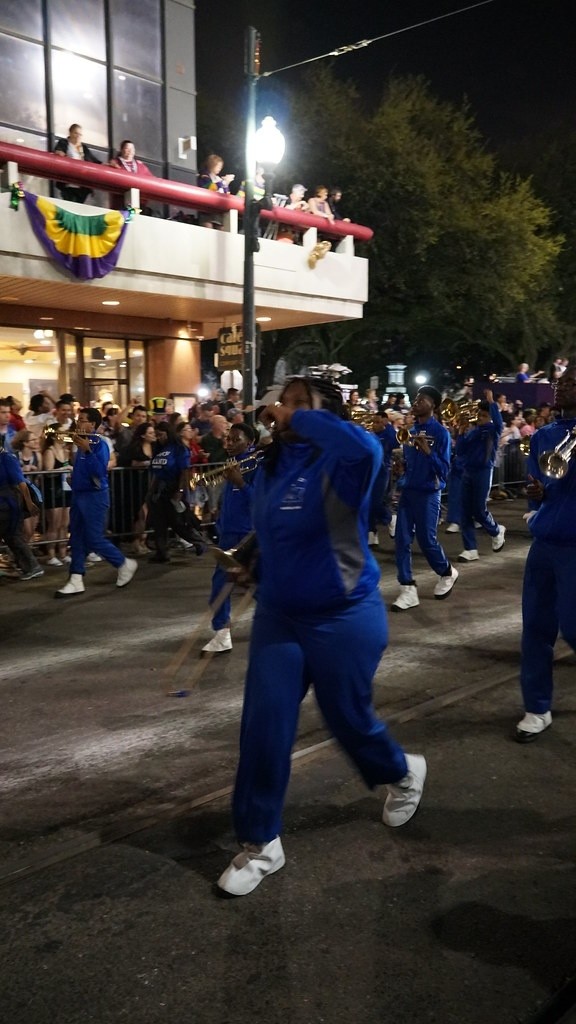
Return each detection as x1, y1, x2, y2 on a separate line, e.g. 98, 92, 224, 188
162, 529, 256, 695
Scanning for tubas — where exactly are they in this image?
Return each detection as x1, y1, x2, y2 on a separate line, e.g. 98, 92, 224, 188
441, 397, 481, 422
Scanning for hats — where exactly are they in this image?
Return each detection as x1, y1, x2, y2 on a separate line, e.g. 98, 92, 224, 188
147, 397, 170, 417
227, 408, 246, 419
388, 411, 405, 422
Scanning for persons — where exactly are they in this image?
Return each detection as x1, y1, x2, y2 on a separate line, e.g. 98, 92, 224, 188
197, 154, 228, 230
201, 423, 260, 653
218, 164, 351, 253
107, 139, 158, 215
0, 359, 576, 567
216, 378, 426, 897
367, 411, 397, 546
0, 434, 45, 581
452, 389, 507, 561
148, 422, 206, 573
55, 124, 109, 204
390, 386, 458, 611
57, 408, 138, 595
516, 362, 576, 746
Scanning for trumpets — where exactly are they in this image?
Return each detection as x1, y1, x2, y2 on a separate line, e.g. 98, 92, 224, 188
44, 424, 101, 445
189, 452, 263, 490
396, 427, 435, 447
348, 408, 374, 430
539, 429, 575, 478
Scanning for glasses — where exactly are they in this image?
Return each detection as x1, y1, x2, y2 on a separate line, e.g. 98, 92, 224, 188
74, 420, 89, 426
551, 380, 576, 391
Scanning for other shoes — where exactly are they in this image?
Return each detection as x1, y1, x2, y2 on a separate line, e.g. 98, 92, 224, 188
432, 564, 459, 600
382, 754, 427, 827
522, 510, 538, 524
388, 515, 397, 539
486, 483, 536, 504
116, 557, 138, 586
56, 574, 85, 596
491, 525, 506, 552
202, 628, 233, 652
517, 711, 552, 735
445, 523, 461, 534
458, 550, 479, 562
0, 510, 222, 581
391, 584, 420, 610
217, 834, 285, 896
367, 531, 379, 546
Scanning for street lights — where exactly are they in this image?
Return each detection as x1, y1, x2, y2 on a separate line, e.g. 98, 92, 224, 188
240, 26, 284, 426
